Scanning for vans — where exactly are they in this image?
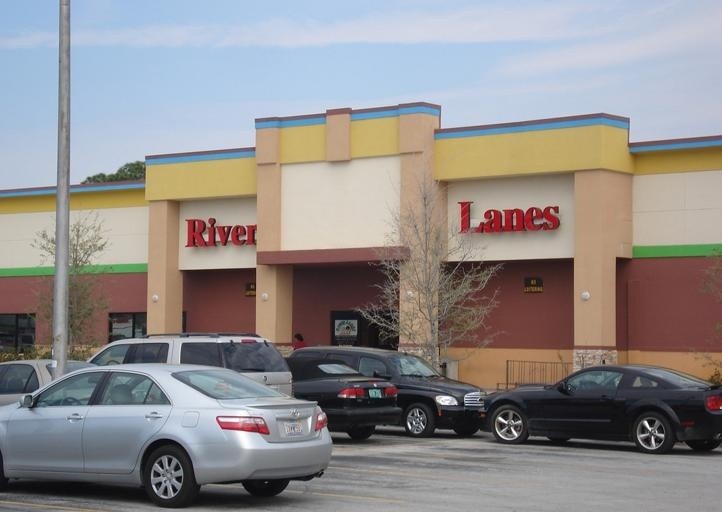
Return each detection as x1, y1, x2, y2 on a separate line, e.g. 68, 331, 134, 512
291, 345, 486, 438
87, 332, 293, 396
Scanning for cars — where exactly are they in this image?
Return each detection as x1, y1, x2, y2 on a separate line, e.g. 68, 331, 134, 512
484, 362, 722, 455
284, 357, 404, 441
0, 358, 101, 406
0, 361, 333, 509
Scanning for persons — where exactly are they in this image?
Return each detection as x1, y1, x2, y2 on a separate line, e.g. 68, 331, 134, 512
293, 333, 304, 350
